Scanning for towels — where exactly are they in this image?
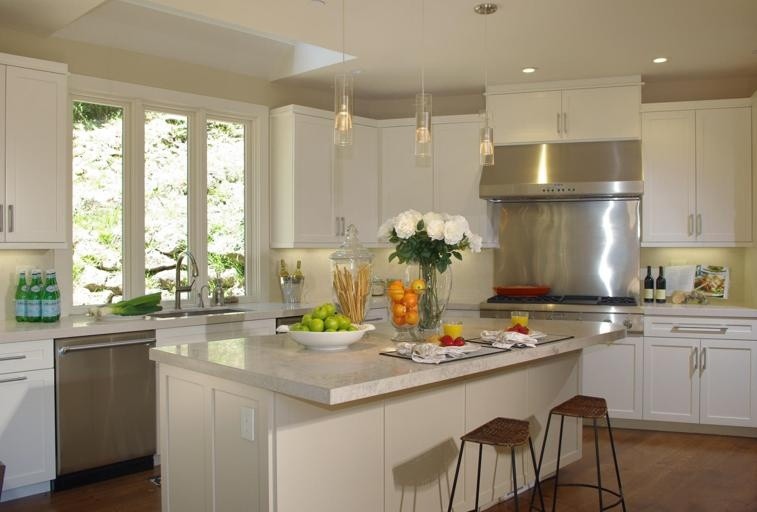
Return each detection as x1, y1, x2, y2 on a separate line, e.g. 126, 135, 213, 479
397, 341, 465, 364
480, 330, 537, 350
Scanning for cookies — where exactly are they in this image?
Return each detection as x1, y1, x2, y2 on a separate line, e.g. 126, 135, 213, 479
332, 264, 369, 324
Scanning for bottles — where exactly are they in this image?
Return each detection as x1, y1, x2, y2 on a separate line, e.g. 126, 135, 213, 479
278, 259, 289, 276
15, 269, 61, 323
655, 266, 666, 304
642, 265, 654, 303
292, 259, 304, 276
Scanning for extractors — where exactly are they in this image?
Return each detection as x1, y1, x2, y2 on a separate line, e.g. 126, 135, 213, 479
478, 141, 644, 202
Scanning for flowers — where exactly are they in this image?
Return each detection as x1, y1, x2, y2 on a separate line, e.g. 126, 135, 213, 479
378, 210, 483, 330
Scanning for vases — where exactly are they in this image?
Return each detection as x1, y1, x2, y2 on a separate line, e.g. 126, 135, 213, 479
401, 262, 452, 332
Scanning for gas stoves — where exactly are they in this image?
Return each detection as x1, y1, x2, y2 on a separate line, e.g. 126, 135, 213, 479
480, 293, 644, 333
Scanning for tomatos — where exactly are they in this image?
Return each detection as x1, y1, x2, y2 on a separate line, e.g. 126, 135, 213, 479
508, 324, 528, 335
442, 334, 466, 346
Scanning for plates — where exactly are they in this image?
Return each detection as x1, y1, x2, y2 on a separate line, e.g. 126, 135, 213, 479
530, 329, 546, 339
451, 341, 480, 352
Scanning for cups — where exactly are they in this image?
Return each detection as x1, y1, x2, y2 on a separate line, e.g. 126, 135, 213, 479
439, 319, 464, 337
510, 310, 529, 327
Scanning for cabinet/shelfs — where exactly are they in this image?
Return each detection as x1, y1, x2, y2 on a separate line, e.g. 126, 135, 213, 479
270, 104, 379, 248
482, 74, 645, 147
0, 340, 55, 492
0, 52, 69, 250
643, 309, 757, 428
641, 98, 752, 247
379, 114, 500, 249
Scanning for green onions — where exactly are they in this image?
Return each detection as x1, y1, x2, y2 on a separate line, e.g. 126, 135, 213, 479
96, 292, 162, 316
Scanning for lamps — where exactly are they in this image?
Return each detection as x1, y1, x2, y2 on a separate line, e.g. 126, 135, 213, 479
334, 0, 353, 144
415, 0, 434, 157
474, 3, 498, 166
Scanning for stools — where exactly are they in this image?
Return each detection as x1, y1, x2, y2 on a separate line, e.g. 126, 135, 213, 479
529, 394, 627, 512
448, 417, 545, 512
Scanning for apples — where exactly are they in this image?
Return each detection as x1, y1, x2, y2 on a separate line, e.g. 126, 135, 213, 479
290, 301, 358, 332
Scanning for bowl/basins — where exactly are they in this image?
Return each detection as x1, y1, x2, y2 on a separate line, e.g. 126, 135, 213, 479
275, 323, 377, 351
493, 285, 552, 296
